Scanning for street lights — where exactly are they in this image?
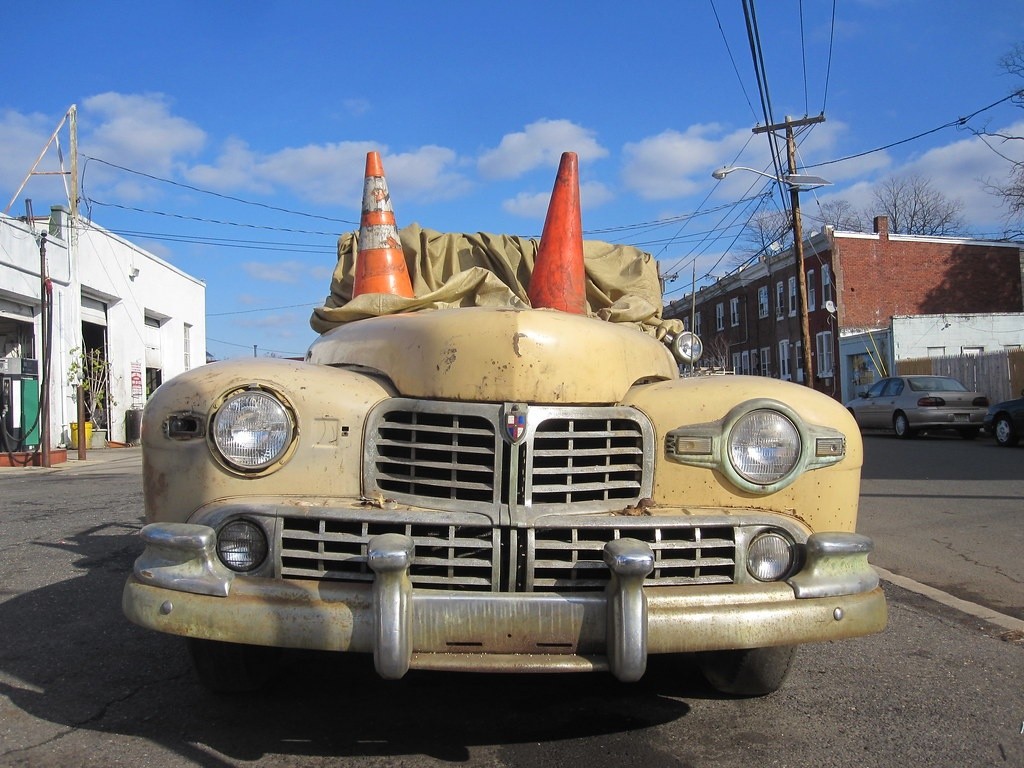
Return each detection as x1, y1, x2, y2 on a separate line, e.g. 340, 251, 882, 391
711, 167, 814, 391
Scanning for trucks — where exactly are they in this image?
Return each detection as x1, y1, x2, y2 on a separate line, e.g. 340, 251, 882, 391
120, 296, 888, 698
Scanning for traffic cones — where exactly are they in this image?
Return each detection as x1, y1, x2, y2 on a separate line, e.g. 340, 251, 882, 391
353, 150, 416, 301
525, 151, 587, 316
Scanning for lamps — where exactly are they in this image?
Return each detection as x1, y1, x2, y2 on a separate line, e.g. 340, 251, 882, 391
130, 268, 140, 278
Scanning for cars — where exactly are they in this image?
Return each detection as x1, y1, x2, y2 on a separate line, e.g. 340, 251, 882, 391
982, 389, 1024, 448
844, 374, 989, 441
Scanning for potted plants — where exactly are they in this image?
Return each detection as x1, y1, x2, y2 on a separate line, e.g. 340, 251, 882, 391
66, 341, 116, 449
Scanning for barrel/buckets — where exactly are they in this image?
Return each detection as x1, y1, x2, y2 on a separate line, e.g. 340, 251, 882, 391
70, 422, 93, 449
